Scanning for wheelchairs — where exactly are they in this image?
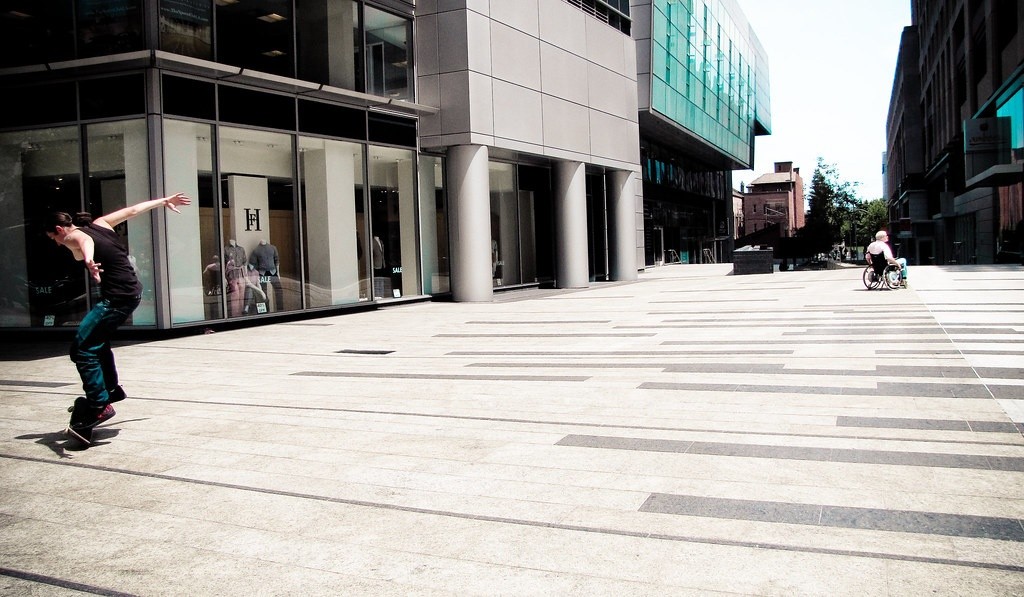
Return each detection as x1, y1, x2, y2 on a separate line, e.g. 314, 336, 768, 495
862, 248, 907, 293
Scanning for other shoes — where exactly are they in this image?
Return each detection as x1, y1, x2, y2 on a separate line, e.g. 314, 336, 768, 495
242, 311, 248, 316
898, 276, 906, 282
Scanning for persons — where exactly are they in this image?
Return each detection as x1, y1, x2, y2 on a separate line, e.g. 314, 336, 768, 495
835, 246, 858, 260
224, 238, 285, 317
46, 192, 192, 425
202, 255, 222, 295
370, 236, 385, 279
492, 235, 499, 277
864, 230, 906, 289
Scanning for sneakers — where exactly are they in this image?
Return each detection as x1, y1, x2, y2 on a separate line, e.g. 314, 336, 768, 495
71, 400, 116, 430
107, 385, 127, 402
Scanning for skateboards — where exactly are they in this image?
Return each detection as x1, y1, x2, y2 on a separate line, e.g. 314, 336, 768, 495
63, 397, 92, 445
